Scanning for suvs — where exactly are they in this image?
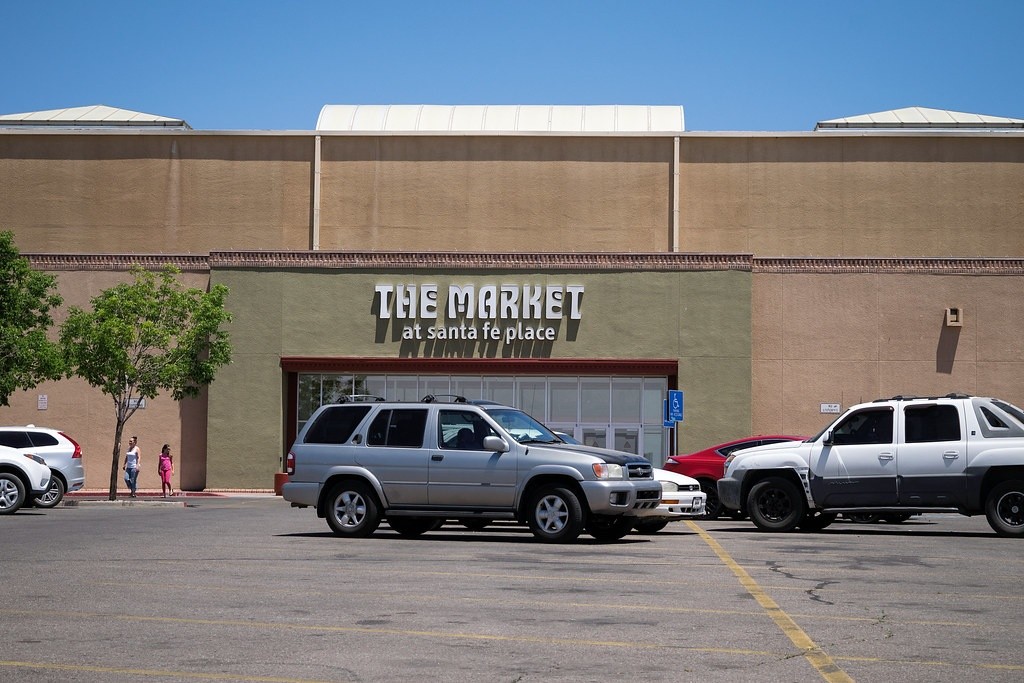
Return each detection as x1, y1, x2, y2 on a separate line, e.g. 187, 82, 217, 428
280, 394, 665, 547
717, 392, 1024, 539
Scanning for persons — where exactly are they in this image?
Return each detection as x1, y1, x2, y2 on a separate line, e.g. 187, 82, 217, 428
122, 436, 141, 497
158, 444, 175, 498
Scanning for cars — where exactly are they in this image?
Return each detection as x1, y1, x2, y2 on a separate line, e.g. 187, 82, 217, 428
661, 434, 816, 520
435, 420, 708, 535
0, 423, 88, 508
0, 445, 54, 516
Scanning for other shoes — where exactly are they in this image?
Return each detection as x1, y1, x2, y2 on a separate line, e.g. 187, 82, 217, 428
130, 490, 133, 495
161, 494, 166, 497
169, 488, 173, 497
129, 494, 136, 498
